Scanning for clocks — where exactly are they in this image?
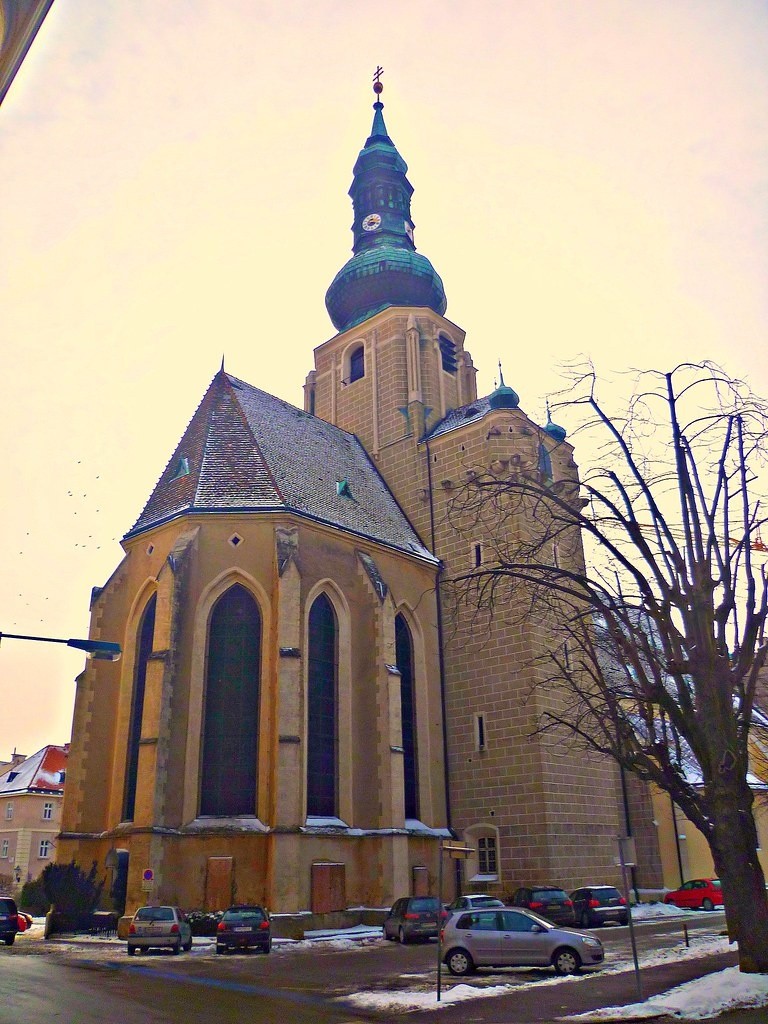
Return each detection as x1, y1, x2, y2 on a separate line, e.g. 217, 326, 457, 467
403, 219, 414, 243
361, 213, 381, 232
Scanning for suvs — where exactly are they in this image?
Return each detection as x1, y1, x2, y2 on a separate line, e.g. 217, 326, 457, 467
508, 884, 577, 925
439, 905, 605, 975
444, 895, 508, 922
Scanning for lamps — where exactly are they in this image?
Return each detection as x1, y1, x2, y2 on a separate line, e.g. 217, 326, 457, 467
14, 865, 21, 881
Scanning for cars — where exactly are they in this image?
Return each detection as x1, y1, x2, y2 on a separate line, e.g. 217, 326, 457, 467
663, 877, 724, 911
17, 909, 32, 932
382, 897, 447, 944
215, 906, 275, 954
570, 886, 630, 926
127, 904, 195, 955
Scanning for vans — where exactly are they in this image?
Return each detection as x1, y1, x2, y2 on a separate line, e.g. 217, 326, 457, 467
0, 897, 20, 946
